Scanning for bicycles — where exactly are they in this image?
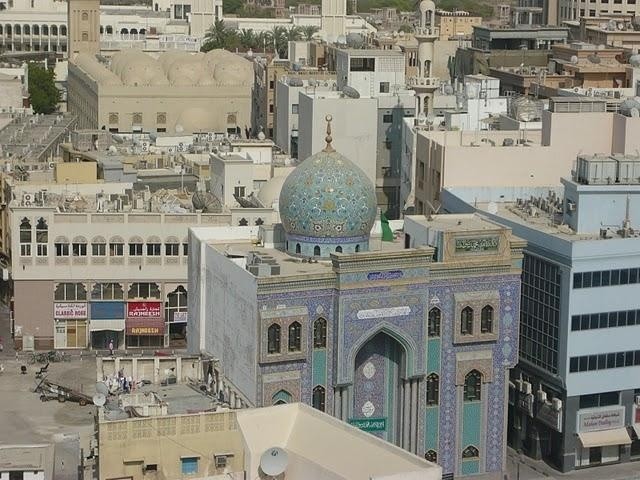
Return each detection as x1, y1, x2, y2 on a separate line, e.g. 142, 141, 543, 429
24, 343, 74, 368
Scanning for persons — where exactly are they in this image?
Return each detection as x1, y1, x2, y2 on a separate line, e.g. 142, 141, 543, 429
118, 368, 124, 380
109, 340, 114, 355
